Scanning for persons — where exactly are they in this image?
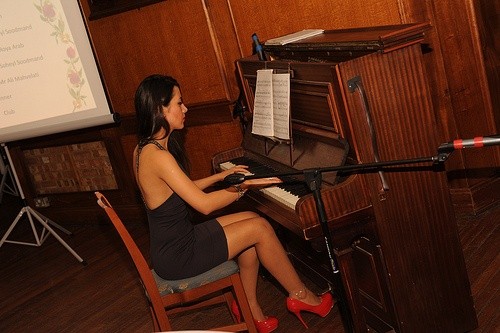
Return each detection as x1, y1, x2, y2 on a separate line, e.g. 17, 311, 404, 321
132, 74, 334, 333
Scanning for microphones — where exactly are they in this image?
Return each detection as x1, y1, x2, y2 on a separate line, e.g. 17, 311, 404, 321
437, 135, 500, 154
252, 33, 268, 62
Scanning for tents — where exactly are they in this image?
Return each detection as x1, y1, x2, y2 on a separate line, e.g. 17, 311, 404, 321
251, 69, 290, 140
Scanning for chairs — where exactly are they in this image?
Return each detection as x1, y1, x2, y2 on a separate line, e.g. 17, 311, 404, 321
94, 191, 257, 333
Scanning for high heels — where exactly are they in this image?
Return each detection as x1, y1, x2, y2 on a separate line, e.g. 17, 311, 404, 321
287, 293, 338, 330
223, 296, 279, 333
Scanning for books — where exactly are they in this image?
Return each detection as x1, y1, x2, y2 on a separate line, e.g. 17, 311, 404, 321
265, 29, 324, 46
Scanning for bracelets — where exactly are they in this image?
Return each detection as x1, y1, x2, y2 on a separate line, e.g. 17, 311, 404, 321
232, 184, 244, 199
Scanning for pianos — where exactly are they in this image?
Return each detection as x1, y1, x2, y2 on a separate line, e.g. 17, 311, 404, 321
210, 21, 480, 333
